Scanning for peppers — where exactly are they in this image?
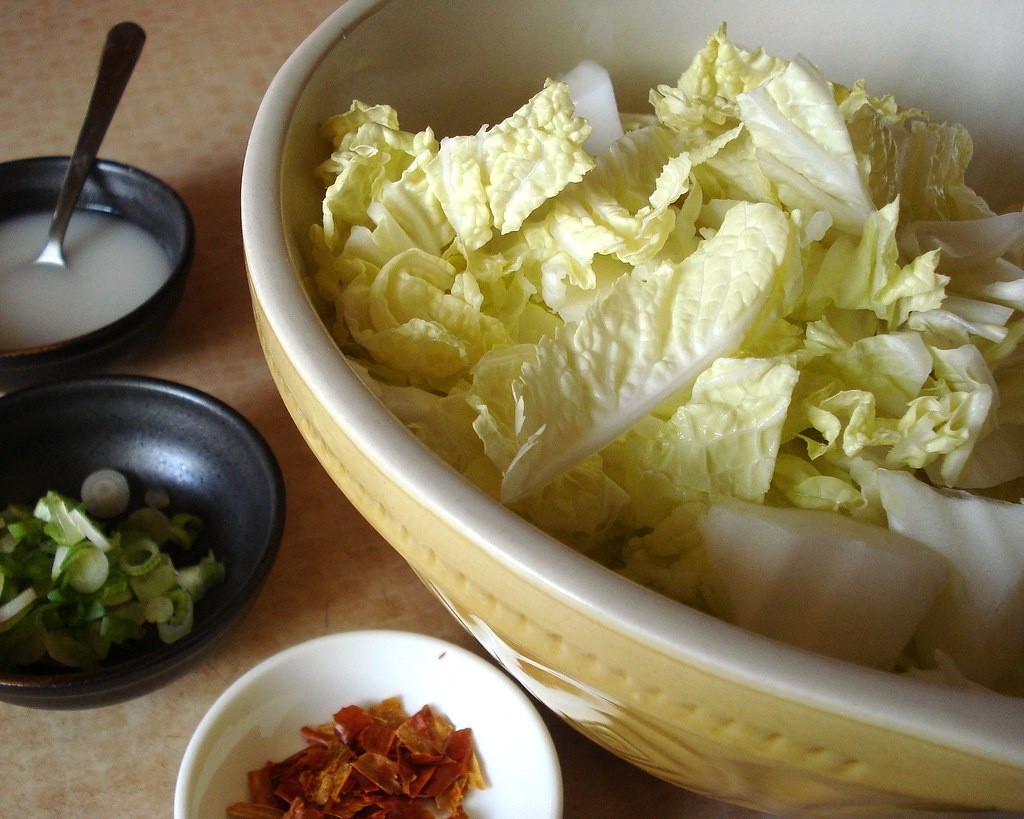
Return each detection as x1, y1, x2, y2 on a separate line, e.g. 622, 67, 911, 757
225, 696, 485, 819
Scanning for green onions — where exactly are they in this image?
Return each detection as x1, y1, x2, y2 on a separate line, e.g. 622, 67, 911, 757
0, 468, 225, 675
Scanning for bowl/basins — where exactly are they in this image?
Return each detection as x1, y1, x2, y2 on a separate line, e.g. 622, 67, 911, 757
0, 375, 287, 710
174, 629, 563, 819
0, 155, 193, 370
239, 0, 1024, 819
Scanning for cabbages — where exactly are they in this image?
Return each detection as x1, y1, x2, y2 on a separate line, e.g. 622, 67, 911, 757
294, 20, 1024, 710
